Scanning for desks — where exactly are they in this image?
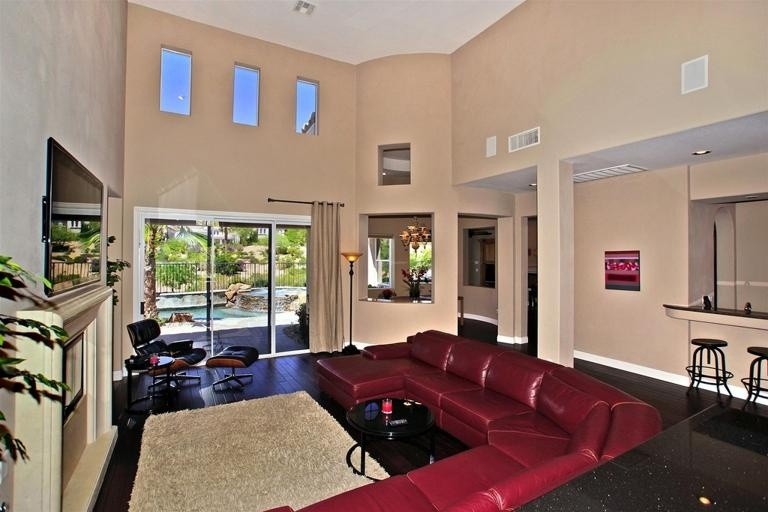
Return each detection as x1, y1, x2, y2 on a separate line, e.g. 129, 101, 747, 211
374, 294, 464, 328
124, 356, 175, 415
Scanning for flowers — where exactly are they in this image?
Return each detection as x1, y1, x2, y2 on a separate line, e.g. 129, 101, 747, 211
400, 264, 433, 287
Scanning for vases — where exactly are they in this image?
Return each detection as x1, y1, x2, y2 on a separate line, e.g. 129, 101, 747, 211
409, 286, 421, 299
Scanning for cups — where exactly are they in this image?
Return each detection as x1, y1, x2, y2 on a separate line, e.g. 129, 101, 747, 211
149, 352, 159, 365
382, 398, 392, 414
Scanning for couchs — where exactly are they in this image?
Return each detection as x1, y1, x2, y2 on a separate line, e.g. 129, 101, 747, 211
265, 326, 661, 511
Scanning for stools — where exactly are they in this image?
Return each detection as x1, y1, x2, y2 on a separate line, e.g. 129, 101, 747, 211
737, 344, 768, 411
685, 337, 735, 401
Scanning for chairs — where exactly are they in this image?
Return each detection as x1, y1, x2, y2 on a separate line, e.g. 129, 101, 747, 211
128, 316, 259, 394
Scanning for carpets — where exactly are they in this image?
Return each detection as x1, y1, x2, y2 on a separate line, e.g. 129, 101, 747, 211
126, 389, 391, 512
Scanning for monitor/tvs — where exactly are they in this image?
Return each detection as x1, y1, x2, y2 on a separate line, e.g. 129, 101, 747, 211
44, 137, 103, 298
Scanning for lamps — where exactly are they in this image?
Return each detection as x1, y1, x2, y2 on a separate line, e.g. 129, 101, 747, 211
399, 212, 432, 256
341, 251, 363, 354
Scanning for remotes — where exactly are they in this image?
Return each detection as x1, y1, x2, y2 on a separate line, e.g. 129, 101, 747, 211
389, 419, 408, 427
